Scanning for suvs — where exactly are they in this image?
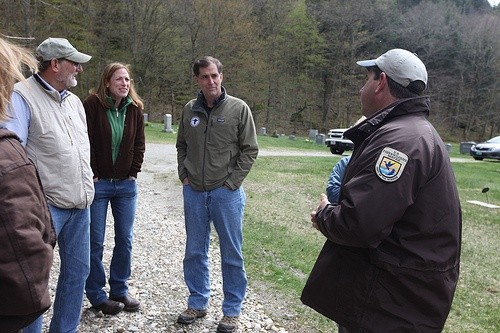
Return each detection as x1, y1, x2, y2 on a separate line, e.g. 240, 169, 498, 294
325, 116, 368, 155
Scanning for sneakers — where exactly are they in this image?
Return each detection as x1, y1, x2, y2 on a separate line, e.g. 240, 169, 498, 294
217, 315, 239, 333
178, 308, 207, 324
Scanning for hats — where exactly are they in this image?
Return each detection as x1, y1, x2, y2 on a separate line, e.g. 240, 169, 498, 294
35, 37, 92, 64
356, 48, 428, 96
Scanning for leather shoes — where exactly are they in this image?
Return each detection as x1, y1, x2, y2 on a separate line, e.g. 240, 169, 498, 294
109, 296, 139, 309
94, 300, 125, 315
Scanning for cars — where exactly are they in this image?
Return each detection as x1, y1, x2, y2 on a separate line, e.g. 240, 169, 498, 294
470, 136, 500, 162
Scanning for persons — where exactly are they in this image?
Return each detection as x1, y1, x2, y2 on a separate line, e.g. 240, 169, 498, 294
177, 56, 259, 332
325, 155, 351, 203
5, 36, 93, 333
0, 37, 57, 333
301, 49, 462, 333
82, 64, 145, 313
